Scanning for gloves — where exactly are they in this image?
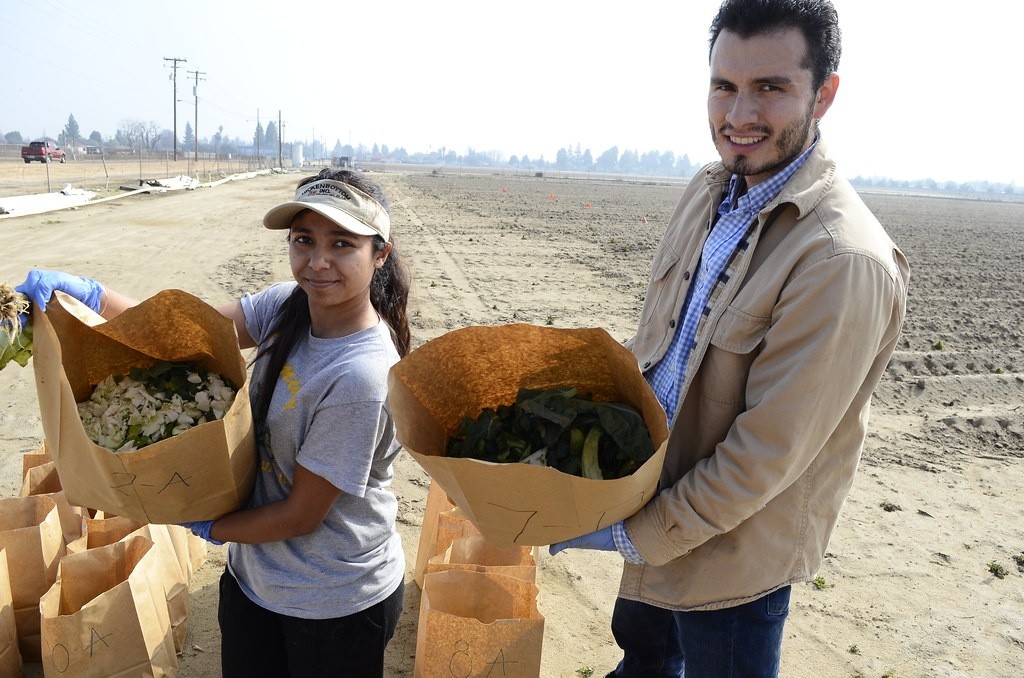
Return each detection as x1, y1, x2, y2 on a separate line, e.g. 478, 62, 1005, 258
180, 520, 226, 545
1, 269, 103, 330
549, 525, 618, 557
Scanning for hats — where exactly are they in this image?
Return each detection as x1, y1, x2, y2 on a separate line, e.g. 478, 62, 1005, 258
263, 179, 390, 243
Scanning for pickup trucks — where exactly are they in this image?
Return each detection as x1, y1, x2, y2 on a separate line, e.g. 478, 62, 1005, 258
21, 141, 67, 164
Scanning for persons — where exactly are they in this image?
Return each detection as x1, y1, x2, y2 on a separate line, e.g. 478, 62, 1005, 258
1, 169, 412, 678
550, 1, 910, 678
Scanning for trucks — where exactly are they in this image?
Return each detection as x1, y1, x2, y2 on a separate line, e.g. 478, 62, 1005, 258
340, 156, 352, 167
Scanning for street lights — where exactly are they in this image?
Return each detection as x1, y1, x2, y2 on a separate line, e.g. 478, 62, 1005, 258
247, 120, 259, 161
177, 99, 198, 162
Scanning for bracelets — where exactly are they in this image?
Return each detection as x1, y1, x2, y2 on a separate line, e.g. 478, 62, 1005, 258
94, 279, 110, 316
